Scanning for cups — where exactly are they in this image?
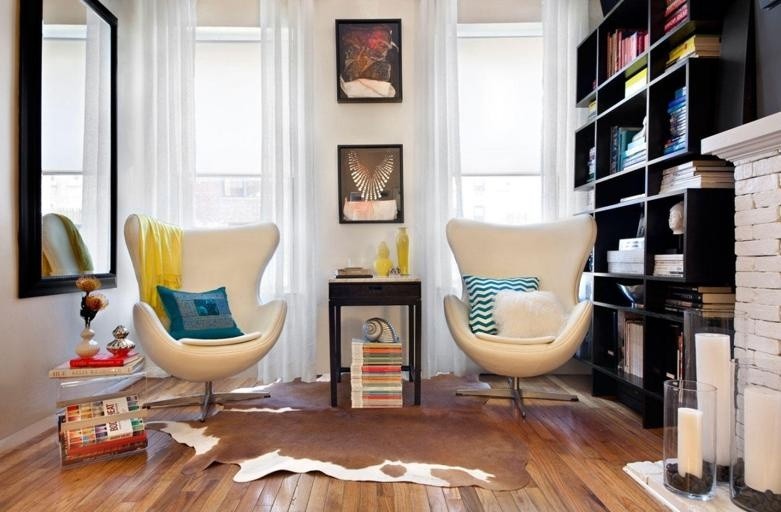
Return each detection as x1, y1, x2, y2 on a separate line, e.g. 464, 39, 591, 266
663, 380, 717, 502
730, 360, 779, 511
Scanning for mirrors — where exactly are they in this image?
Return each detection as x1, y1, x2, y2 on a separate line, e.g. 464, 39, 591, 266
18, 0, 118, 299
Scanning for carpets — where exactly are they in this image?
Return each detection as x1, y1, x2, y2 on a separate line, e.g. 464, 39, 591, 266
145, 374, 530, 491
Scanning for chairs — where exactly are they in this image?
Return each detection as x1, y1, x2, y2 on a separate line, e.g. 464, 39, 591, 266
124, 214, 287, 422
41, 213, 80, 276
443, 213, 599, 418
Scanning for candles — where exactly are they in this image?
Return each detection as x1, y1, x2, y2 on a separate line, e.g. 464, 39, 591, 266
693, 332, 734, 467
744, 387, 781, 494
677, 407, 705, 479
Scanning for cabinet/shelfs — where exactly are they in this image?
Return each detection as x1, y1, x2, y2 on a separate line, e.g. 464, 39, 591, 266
49, 356, 149, 472
574, 0, 758, 430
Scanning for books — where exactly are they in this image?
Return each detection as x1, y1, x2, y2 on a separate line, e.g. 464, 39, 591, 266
333, 268, 373, 277
663, 284, 736, 319
606, 0, 735, 196
69, 352, 141, 366
584, 147, 597, 182
653, 253, 684, 278
48, 355, 145, 375
57, 394, 149, 470
349, 337, 403, 409
623, 317, 644, 379
333, 275, 376, 278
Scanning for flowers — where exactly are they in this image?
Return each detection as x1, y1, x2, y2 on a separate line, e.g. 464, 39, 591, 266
76, 276, 109, 328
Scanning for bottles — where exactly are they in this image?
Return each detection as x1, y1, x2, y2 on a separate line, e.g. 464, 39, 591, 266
396, 227, 410, 276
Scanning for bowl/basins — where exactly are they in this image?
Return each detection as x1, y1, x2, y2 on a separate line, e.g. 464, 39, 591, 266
617, 280, 645, 310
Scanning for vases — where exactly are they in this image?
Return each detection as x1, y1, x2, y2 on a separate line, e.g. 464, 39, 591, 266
107, 325, 135, 357
374, 241, 392, 277
75, 328, 99, 357
397, 227, 409, 275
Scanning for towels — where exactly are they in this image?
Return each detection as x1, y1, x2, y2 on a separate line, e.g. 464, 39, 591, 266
41, 214, 94, 277
134, 214, 181, 327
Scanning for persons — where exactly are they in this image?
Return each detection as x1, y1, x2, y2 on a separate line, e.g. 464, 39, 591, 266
668, 201, 684, 235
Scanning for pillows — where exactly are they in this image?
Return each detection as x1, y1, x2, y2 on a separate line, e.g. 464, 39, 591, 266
463, 274, 540, 335
157, 286, 244, 340
493, 289, 563, 340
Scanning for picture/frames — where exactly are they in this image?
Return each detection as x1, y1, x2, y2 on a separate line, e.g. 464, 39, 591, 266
336, 144, 403, 223
335, 18, 402, 103
